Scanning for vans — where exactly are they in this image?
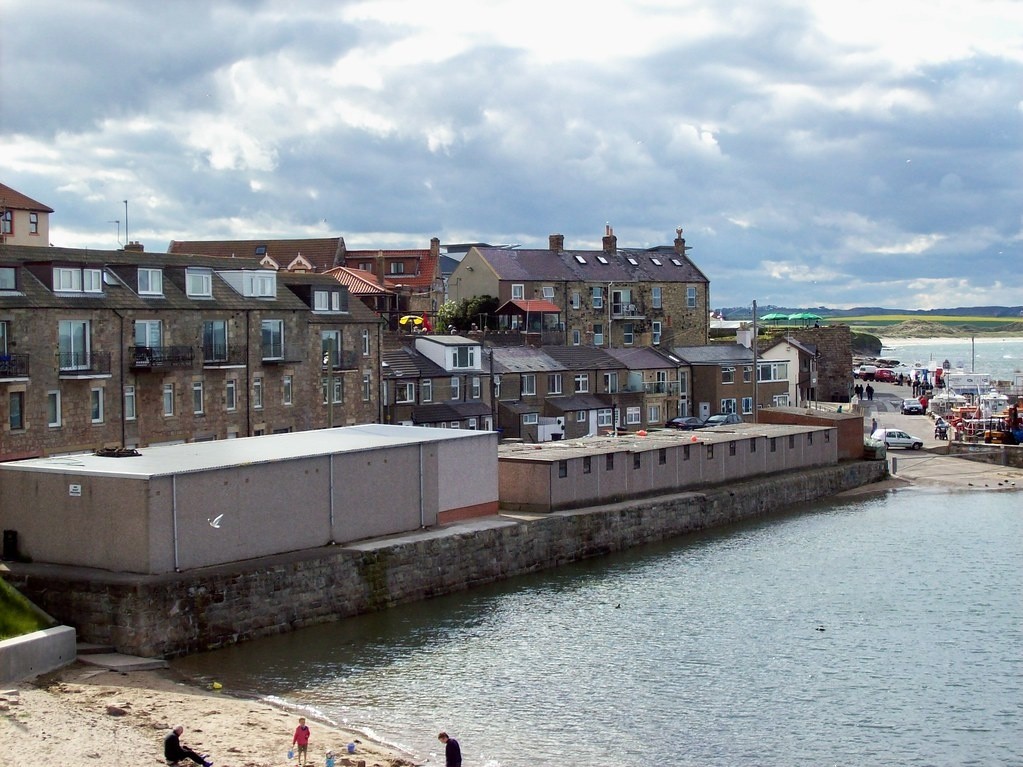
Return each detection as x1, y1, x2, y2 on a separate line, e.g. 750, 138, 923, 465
859, 365, 879, 373
874, 369, 898, 382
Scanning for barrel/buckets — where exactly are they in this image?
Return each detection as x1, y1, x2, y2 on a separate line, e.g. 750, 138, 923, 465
348, 743, 356, 754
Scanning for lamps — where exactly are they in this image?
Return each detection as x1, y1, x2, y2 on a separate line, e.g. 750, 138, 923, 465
465, 266, 473, 272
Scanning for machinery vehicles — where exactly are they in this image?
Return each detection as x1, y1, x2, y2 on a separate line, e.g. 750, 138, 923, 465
984, 414, 1020, 446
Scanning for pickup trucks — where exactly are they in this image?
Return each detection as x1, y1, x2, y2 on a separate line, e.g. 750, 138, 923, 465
860, 373, 876, 382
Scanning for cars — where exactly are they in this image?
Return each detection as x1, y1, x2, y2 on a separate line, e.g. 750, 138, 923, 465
853, 372, 859, 379
663, 416, 704, 430
900, 399, 923, 414
872, 427, 924, 450
703, 412, 742, 427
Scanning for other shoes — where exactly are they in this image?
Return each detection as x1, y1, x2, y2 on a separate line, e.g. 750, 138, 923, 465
200, 755, 206, 759
203, 761, 214, 767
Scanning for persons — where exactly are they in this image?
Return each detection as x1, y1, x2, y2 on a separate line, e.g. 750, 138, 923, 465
870, 418, 878, 437
899, 373, 903, 386
436, 731, 462, 767
935, 418, 942, 439
837, 405, 842, 413
908, 374, 911, 386
292, 716, 311, 766
854, 383, 874, 402
920, 396, 928, 415
163, 726, 214, 767
